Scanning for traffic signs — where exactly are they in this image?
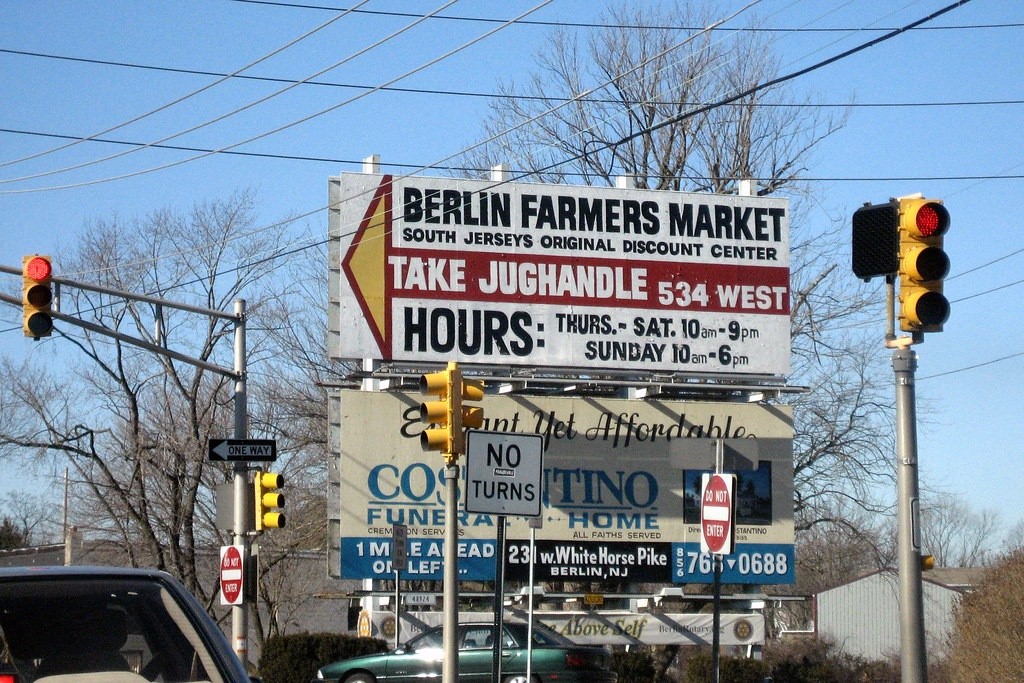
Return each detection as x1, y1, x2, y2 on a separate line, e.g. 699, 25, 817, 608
208, 438, 277, 461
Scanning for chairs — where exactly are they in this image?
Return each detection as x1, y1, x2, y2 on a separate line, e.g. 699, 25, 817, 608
465, 639, 476, 648
34, 609, 129, 680
485, 635, 492, 647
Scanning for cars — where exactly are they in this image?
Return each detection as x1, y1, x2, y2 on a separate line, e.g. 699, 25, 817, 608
310, 620, 619, 683
0, 564, 252, 683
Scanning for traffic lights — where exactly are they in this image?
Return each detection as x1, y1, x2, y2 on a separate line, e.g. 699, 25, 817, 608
456, 369, 485, 452
898, 197, 951, 332
419, 371, 452, 456
255, 471, 286, 531
851, 203, 898, 278
22, 255, 53, 338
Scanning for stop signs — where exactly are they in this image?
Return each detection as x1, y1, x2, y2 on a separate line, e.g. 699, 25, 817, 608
220, 545, 244, 604
701, 473, 733, 555
